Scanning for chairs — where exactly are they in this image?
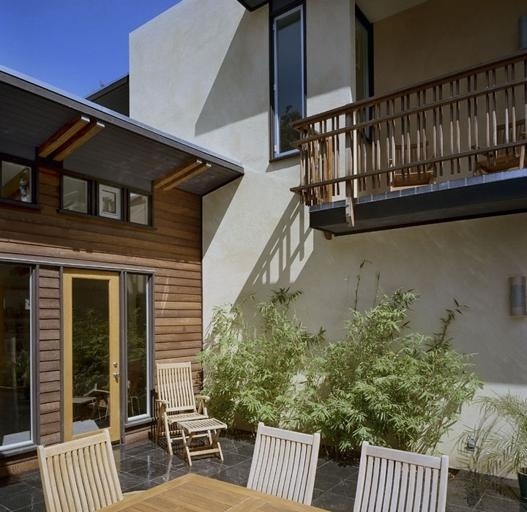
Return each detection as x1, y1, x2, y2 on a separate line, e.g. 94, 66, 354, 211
386, 140, 431, 190
155, 361, 210, 455
353, 441, 449, 512
72, 373, 140, 428
467, 119, 525, 177
36, 427, 124, 511
247, 421, 321, 506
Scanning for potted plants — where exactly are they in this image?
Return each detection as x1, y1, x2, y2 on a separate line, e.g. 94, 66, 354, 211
453, 392, 527, 512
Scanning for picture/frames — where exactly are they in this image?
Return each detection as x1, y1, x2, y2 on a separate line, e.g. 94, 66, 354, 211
96, 179, 125, 224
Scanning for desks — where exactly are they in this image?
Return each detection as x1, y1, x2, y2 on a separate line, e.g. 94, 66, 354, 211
96, 472, 327, 511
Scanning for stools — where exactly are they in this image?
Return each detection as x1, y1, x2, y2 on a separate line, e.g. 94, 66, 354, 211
177, 418, 227, 467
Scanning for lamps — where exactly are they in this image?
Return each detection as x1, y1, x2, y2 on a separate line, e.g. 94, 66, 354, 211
507, 275, 527, 320
